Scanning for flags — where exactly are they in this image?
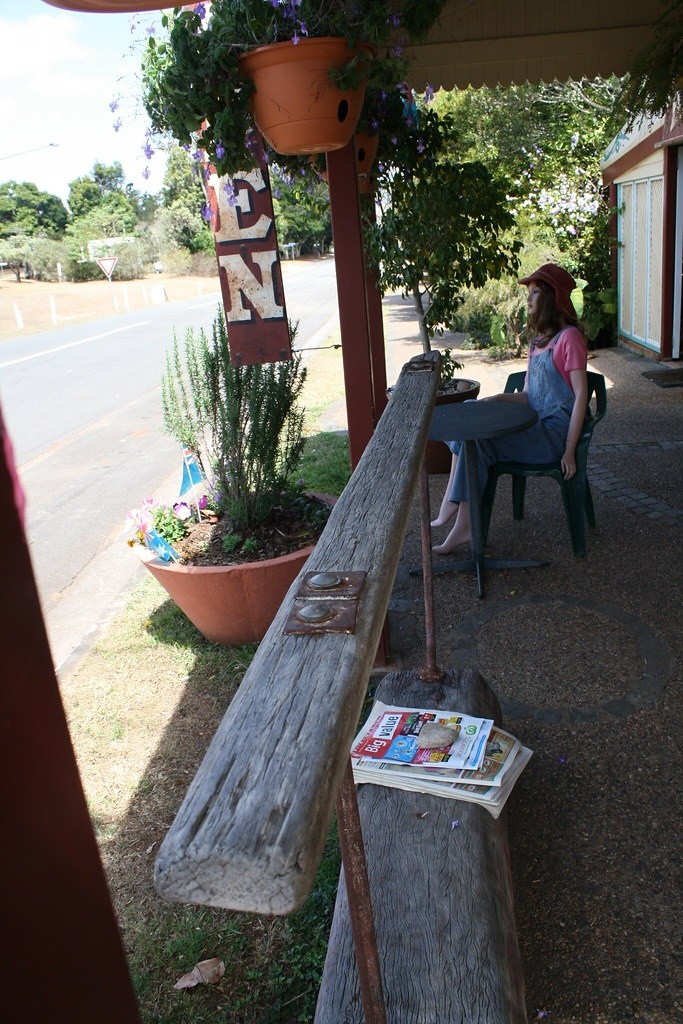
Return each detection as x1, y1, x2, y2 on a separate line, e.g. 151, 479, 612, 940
178, 445, 203, 496
143, 530, 180, 561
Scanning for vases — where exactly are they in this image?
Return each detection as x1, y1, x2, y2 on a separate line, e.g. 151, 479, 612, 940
239, 36, 368, 156
307, 133, 378, 194
140, 491, 336, 648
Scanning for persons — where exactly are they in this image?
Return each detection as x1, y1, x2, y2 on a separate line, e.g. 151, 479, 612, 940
428, 264, 589, 553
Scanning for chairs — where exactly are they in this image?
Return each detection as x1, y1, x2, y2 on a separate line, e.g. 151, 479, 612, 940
505, 371, 607, 558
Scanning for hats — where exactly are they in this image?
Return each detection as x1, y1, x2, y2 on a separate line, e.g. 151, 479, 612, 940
518, 263, 578, 320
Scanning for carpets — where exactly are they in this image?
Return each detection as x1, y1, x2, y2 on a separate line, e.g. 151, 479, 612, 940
641, 368, 683, 388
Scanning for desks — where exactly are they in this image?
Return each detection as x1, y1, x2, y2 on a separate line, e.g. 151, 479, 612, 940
409, 400, 553, 601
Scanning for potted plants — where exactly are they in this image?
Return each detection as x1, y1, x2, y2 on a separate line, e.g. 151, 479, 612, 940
357, 160, 525, 475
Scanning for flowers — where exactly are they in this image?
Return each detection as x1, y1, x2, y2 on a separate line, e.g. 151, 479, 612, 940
124, 302, 311, 560
109, 0, 432, 221
268, 94, 461, 207
